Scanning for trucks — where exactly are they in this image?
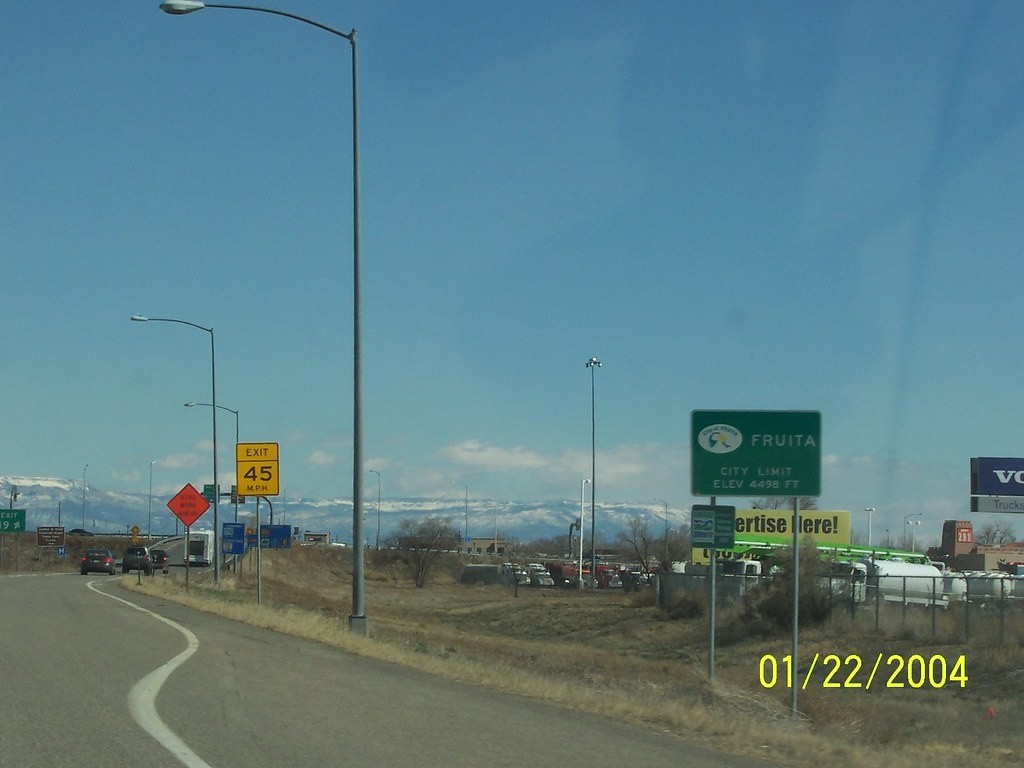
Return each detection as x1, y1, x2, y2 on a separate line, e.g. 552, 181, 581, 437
184, 530, 214, 566
817, 558, 949, 609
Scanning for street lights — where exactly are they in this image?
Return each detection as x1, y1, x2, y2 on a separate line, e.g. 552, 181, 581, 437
83, 464, 89, 529
655, 497, 668, 562
585, 357, 603, 588
579, 479, 590, 581
129, 315, 221, 584
149, 460, 161, 539
483, 503, 498, 554
903, 513, 923, 551
865, 508, 875, 548
450, 481, 468, 538
906, 521, 921, 552
370, 469, 381, 548
184, 402, 239, 573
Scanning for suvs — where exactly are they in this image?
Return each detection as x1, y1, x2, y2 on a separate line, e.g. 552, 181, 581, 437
150, 549, 168, 574
122, 547, 154, 576
81, 547, 115, 575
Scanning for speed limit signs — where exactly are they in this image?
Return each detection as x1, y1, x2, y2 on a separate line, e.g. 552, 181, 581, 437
235, 442, 279, 496
